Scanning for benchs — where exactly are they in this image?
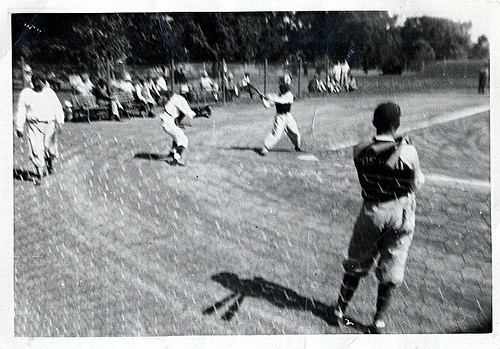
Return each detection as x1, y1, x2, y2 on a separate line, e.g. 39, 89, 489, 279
70, 73, 248, 125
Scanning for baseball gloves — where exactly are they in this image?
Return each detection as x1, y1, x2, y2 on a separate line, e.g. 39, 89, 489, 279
199, 104, 211, 119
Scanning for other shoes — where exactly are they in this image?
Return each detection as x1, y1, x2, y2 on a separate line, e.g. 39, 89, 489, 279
148, 111, 155, 115
362, 323, 386, 334
296, 147, 306, 152
46, 159, 56, 174
261, 146, 267, 155
35, 173, 44, 184
173, 158, 185, 166
117, 116, 123, 123
329, 305, 342, 324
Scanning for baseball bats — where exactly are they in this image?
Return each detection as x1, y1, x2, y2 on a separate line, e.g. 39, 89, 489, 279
384, 133, 410, 172
242, 79, 269, 101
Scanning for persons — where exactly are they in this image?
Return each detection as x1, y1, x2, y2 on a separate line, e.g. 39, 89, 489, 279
259, 83, 308, 156
159, 84, 200, 166
479, 63, 488, 94
322, 101, 425, 334
14, 71, 64, 186
242, 72, 250, 94
302, 60, 308, 77
276, 64, 292, 85
73, 68, 192, 122
201, 71, 220, 104
308, 56, 358, 93
224, 72, 241, 102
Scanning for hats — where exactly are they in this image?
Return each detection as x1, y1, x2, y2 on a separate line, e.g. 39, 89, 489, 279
278, 73, 293, 87
32, 72, 46, 79
180, 84, 192, 94
